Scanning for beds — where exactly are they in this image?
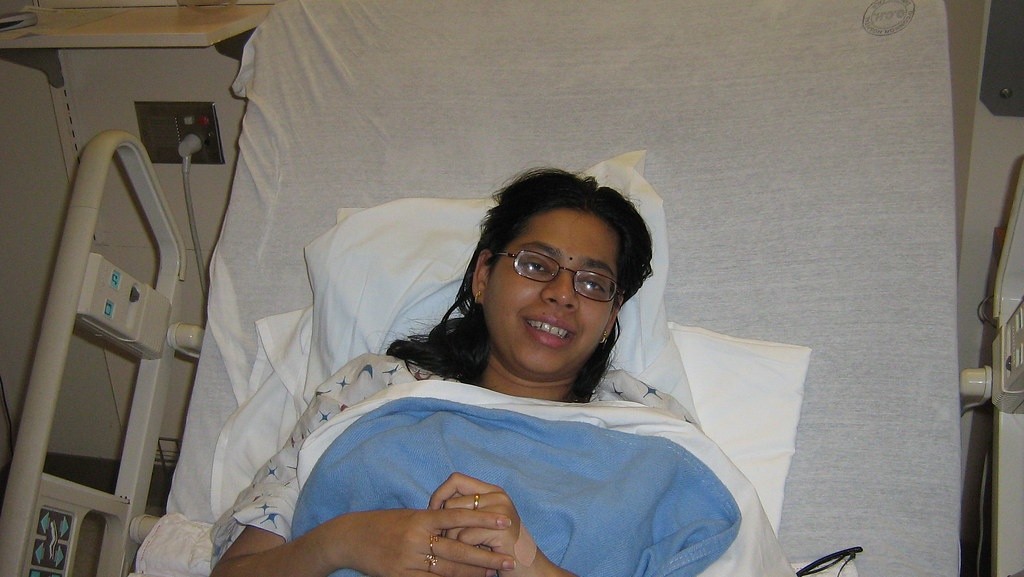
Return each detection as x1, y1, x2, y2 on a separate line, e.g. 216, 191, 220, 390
0, 0, 1024, 577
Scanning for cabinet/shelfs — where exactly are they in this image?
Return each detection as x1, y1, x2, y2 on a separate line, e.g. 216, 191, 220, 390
0, 0, 273, 475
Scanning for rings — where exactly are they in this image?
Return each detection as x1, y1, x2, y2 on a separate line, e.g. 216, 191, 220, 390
430, 534, 439, 555
473, 494, 479, 509
424, 554, 438, 572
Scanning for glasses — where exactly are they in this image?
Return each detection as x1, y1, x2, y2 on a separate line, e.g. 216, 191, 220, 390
495, 250, 624, 303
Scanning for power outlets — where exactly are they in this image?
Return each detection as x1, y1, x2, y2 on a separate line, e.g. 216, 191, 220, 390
135, 100, 223, 165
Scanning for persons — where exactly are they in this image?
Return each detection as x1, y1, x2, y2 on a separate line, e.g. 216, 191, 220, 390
209, 169, 801, 577
